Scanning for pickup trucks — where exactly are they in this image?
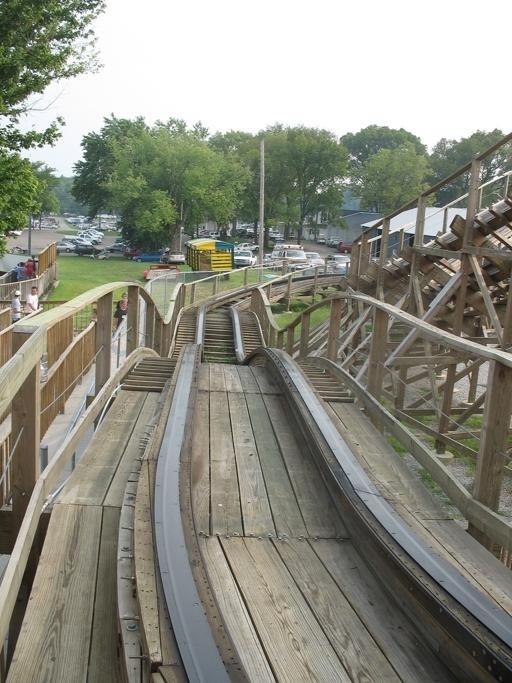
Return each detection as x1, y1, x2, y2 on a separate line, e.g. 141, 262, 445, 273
165, 251, 185, 264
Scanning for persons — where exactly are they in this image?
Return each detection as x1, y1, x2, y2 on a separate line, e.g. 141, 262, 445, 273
11, 261, 28, 282
114, 290, 129, 329
24, 286, 39, 315
31, 254, 37, 278
25, 256, 33, 279
12, 289, 24, 324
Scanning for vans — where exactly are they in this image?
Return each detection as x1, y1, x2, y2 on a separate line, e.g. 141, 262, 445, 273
269, 243, 310, 272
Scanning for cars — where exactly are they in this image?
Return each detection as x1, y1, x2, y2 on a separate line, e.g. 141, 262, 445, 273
44, 224, 58, 229
8, 230, 22, 236
198, 224, 280, 266
305, 236, 352, 274
56, 221, 163, 263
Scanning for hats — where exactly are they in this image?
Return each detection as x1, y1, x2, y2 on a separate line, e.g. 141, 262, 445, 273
14, 290, 21, 295
